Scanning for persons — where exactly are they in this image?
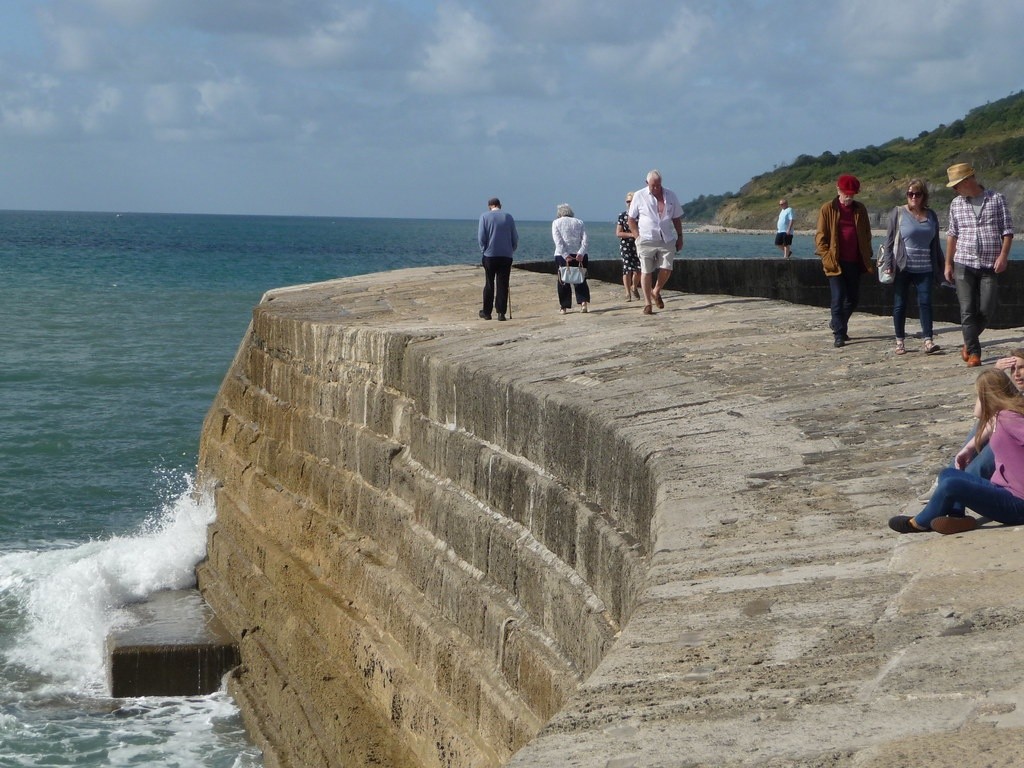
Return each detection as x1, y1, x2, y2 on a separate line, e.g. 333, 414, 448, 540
887, 347, 1024, 537
775, 199, 794, 260
883, 179, 944, 355
477, 197, 518, 321
815, 174, 873, 347
944, 163, 1014, 366
616, 170, 684, 314
552, 204, 590, 315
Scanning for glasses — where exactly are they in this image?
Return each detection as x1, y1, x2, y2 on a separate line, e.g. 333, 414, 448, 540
906, 191, 921, 199
626, 200, 631, 203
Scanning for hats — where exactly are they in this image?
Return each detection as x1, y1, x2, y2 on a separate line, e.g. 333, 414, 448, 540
838, 175, 861, 195
488, 198, 500, 205
945, 163, 977, 187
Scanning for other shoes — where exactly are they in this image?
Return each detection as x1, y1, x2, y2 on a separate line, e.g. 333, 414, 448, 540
930, 515, 977, 535
915, 476, 940, 503
968, 354, 981, 367
962, 344, 968, 362
889, 515, 930, 534
651, 290, 664, 308
625, 294, 631, 302
479, 310, 492, 320
829, 319, 848, 341
580, 302, 587, 312
631, 286, 640, 300
643, 305, 652, 315
834, 336, 845, 347
557, 308, 566, 315
498, 313, 506, 321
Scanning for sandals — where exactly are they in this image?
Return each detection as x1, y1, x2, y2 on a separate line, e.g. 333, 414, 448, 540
924, 342, 940, 354
895, 342, 906, 355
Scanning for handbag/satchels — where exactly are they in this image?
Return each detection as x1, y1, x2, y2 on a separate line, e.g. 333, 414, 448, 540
876, 206, 903, 285
558, 258, 587, 285
